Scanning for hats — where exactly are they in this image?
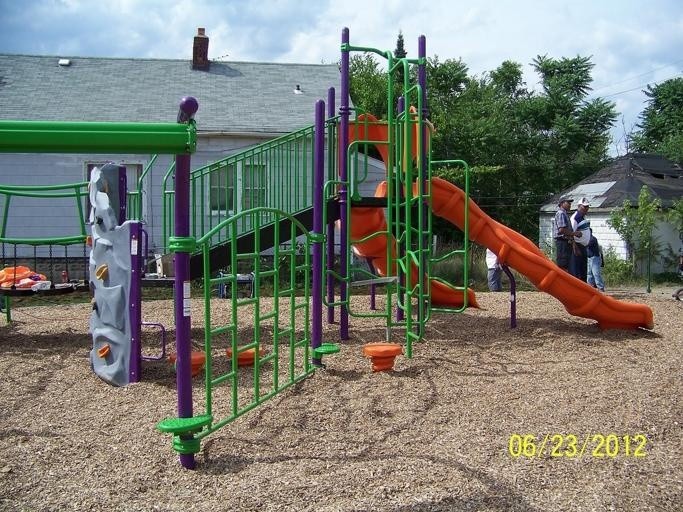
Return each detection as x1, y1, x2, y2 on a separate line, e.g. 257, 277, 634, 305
578, 197, 591, 207
558, 194, 573, 207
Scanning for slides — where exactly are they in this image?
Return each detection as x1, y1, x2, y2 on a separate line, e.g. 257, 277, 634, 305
335, 181, 483, 310
409, 177, 655, 331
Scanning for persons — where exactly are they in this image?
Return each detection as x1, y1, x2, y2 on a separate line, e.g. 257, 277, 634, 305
586, 228, 604, 292
570, 197, 591, 283
485, 247, 503, 291
554, 194, 582, 270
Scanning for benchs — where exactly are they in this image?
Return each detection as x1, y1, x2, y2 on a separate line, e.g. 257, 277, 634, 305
219, 272, 254, 299
